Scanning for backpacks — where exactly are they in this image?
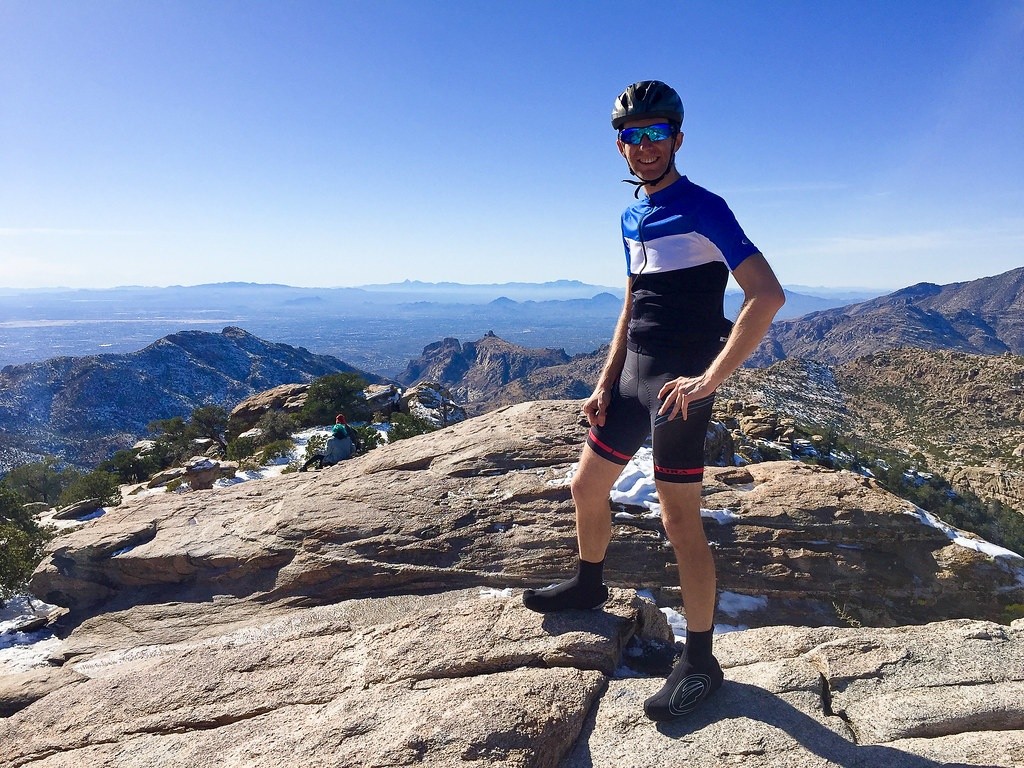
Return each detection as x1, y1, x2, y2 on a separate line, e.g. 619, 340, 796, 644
349, 427, 359, 451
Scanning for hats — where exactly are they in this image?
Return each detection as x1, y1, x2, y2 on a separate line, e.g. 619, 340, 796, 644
336, 414, 345, 423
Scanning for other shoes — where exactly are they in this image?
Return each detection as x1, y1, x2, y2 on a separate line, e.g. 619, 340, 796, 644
315, 466, 323, 470
643, 624, 724, 721
297, 466, 308, 472
522, 556, 609, 613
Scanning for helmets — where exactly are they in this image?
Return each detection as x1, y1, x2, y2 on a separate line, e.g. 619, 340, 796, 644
611, 80, 684, 129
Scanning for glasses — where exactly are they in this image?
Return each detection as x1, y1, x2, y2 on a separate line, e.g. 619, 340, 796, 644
618, 123, 677, 145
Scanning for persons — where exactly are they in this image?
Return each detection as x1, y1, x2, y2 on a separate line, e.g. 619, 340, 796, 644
524, 80, 786, 721
298, 414, 358, 473
